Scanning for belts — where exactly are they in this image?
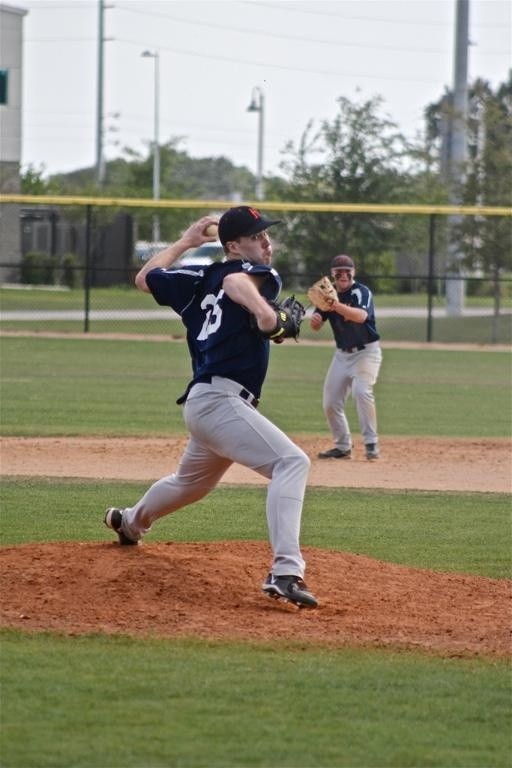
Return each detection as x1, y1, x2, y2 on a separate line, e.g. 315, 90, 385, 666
198, 374, 259, 408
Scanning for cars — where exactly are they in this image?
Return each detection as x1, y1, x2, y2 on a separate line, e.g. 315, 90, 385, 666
134, 240, 169, 258
179, 242, 228, 268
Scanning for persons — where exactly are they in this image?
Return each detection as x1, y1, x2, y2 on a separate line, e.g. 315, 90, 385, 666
103, 203, 322, 609
305, 256, 383, 462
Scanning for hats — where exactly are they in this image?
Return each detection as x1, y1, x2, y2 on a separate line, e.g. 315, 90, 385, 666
331, 255, 355, 271
219, 206, 281, 244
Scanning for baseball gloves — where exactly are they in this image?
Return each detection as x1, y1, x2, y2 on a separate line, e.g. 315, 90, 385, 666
277, 295, 303, 344
307, 276, 338, 311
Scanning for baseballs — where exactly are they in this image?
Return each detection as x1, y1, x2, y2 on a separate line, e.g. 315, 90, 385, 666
205, 224, 219, 235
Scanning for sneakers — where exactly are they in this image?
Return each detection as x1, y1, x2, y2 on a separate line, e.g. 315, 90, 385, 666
103, 506, 142, 546
365, 444, 380, 462
318, 448, 352, 460
261, 572, 318, 610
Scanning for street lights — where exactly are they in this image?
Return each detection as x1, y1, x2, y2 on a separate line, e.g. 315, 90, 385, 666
246, 84, 264, 203
139, 48, 162, 259
